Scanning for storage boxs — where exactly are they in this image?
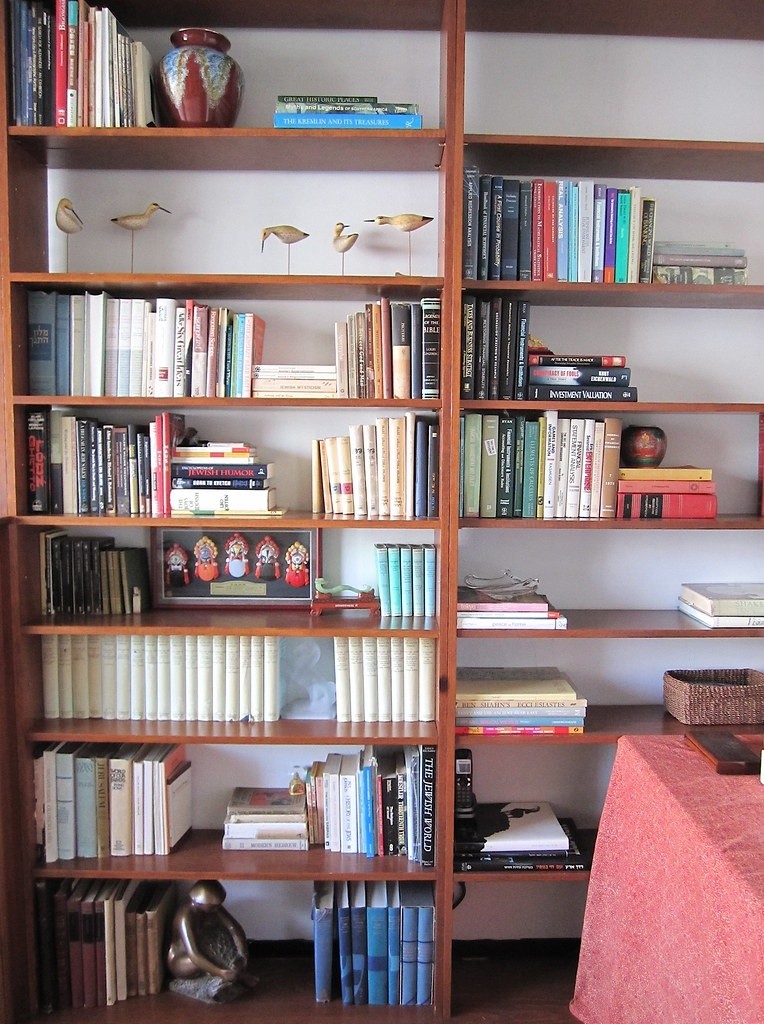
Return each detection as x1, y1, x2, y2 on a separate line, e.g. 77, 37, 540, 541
615, 482, 717, 520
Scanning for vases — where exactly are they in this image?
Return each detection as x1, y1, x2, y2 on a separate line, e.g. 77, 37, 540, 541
149, 29, 241, 127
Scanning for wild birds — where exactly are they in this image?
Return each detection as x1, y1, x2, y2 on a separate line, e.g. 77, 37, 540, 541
362, 213, 434, 276
260, 224, 310, 274
106, 203, 173, 273
331, 222, 359, 276
55, 198, 84, 272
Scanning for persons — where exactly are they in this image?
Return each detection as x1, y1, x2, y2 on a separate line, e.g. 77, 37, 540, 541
165, 880, 260, 990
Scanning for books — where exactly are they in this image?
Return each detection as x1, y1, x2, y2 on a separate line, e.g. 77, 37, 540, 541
453, 802, 586, 872
35, 880, 179, 1013
377, 542, 436, 616
31, 291, 440, 400
28, 411, 289, 516
334, 636, 435, 721
40, 530, 150, 616
454, 666, 587, 735
459, 412, 718, 517
221, 746, 435, 866
311, 880, 436, 1006
34, 742, 193, 863
312, 412, 439, 518
43, 635, 280, 721
461, 166, 749, 401
10, 0, 159, 128
457, 586, 568, 629
676, 583, 763, 628
274, 96, 422, 129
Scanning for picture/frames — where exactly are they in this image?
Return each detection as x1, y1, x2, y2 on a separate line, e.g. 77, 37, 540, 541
148, 528, 322, 608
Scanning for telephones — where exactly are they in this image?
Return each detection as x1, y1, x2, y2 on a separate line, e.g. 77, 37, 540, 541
454, 747, 477, 821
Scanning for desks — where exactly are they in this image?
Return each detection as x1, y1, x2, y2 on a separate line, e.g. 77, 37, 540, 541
567, 734, 764, 1024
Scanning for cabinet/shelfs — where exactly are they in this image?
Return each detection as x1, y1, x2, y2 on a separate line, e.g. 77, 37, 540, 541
0, 0, 446, 1024
446, 1, 764, 1024
